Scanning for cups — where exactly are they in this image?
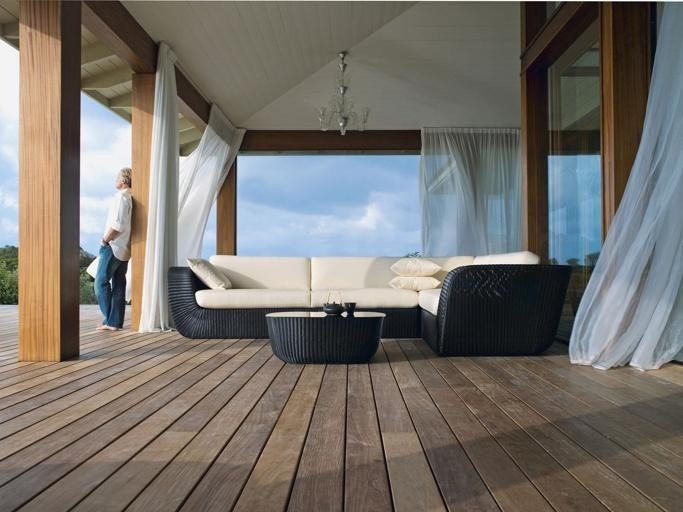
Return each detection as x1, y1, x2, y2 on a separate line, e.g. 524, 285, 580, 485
344, 302, 356, 316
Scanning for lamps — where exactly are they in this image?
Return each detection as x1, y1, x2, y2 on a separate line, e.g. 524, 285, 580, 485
318, 48, 370, 137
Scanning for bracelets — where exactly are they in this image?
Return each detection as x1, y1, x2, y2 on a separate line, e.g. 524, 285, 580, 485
100, 237, 109, 246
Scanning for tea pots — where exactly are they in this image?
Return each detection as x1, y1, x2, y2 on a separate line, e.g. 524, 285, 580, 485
323, 300, 343, 316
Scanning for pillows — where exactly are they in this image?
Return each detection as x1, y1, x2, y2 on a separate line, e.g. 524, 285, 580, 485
388, 254, 442, 291
185, 253, 233, 291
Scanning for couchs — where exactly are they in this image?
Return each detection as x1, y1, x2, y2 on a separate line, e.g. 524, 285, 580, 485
167, 252, 570, 363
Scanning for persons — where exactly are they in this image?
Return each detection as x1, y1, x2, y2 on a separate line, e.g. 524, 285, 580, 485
92, 168, 132, 332
